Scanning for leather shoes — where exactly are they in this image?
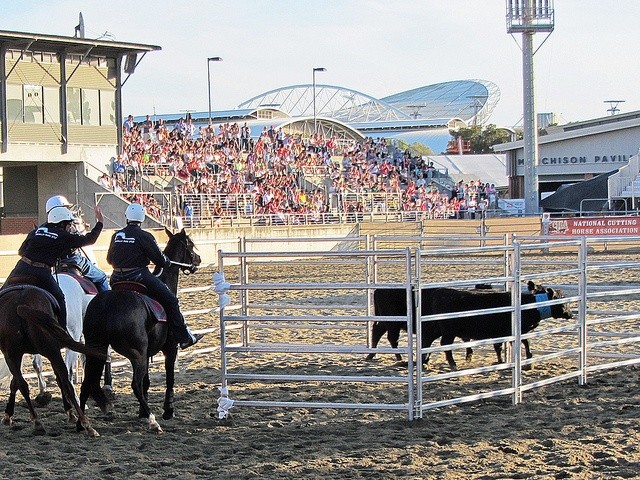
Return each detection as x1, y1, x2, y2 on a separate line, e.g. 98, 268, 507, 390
180, 333, 203, 350
105, 357, 111, 363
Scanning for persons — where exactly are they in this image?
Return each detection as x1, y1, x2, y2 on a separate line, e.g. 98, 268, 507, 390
0, 206, 103, 333
45, 195, 110, 291
99, 116, 497, 228
107, 204, 203, 349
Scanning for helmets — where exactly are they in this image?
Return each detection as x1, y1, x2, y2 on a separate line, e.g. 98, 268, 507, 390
125, 204, 145, 222
46, 196, 73, 213
48, 207, 78, 224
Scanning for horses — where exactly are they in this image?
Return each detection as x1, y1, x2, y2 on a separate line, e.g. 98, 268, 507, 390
75, 226, 203, 435
363, 282, 511, 365
29, 202, 119, 415
1, 219, 98, 441
410, 283, 574, 372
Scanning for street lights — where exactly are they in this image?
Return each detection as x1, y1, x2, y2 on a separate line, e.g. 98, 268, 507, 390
207, 57, 222, 125
313, 66, 328, 134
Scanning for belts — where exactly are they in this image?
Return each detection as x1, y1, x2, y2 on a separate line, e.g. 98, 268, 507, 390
21, 257, 50, 268
113, 267, 139, 273
57, 253, 76, 259
